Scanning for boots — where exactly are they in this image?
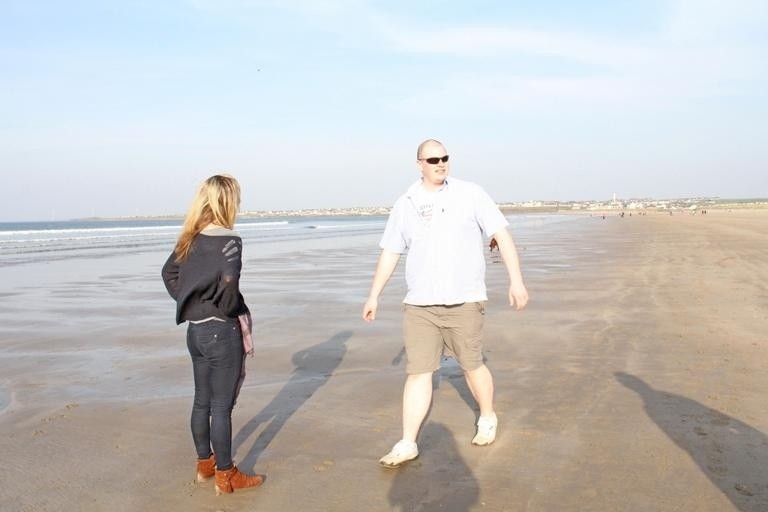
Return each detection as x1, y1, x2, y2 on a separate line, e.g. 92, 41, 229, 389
197, 456, 264, 494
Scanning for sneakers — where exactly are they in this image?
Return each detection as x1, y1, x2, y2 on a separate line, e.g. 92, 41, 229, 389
472, 412, 498, 445
378, 439, 419, 469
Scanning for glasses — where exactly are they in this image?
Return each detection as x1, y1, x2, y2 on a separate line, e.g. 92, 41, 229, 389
420, 155, 449, 164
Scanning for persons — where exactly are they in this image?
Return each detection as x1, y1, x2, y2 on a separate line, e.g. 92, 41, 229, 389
160, 174, 267, 494
361, 138, 530, 466
